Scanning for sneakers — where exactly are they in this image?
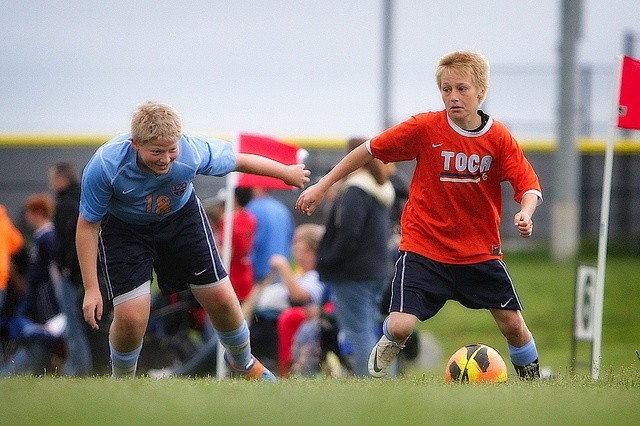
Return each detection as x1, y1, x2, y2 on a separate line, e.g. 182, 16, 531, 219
368, 336, 404, 377
223, 351, 276, 381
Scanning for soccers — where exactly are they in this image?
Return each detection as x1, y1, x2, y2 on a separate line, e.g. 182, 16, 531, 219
448, 342, 508, 381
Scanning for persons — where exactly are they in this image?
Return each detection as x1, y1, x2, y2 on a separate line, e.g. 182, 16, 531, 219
218, 137, 420, 380
48, 159, 82, 291
0, 202, 25, 325
295, 50, 545, 383
22, 189, 65, 373
73, 101, 312, 386
